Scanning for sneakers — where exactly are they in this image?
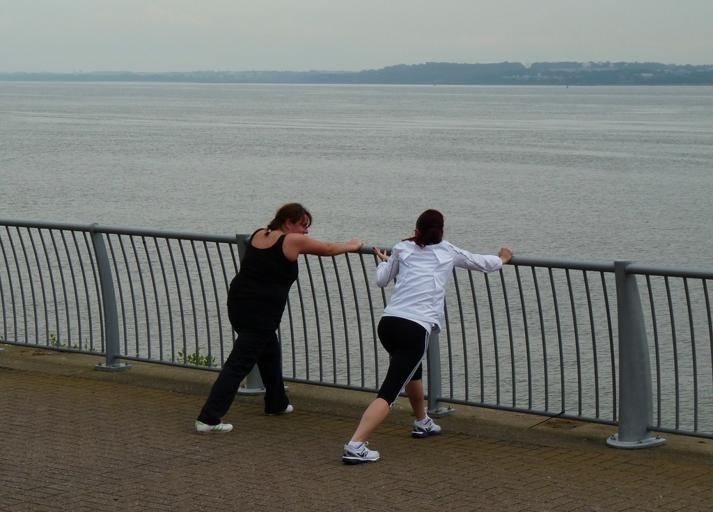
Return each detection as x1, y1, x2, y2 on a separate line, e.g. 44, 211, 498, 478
342, 440, 382, 464
412, 415, 441, 438
194, 419, 233, 435
283, 403, 294, 414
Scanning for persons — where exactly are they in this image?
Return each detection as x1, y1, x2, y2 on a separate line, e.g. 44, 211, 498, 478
195, 203, 363, 435
340, 209, 513, 464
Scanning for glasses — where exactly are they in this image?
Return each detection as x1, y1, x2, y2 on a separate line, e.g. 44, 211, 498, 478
299, 222, 308, 230
